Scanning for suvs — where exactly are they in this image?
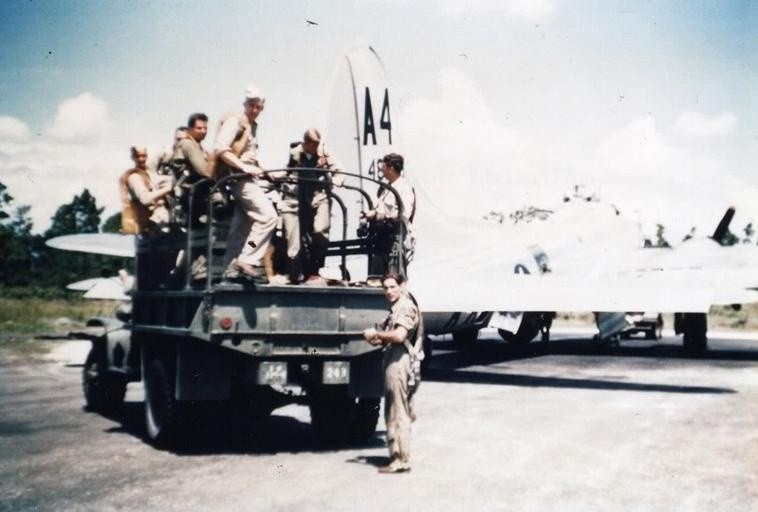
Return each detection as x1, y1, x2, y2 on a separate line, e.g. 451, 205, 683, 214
28, 166, 408, 448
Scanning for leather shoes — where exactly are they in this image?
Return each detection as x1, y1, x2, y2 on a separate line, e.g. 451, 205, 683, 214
375, 453, 413, 473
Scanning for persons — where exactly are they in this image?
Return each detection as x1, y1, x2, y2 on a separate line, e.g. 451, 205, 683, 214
215, 87, 282, 286
117, 112, 338, 287
363, 272, 425, 475
359, 151, 415, 288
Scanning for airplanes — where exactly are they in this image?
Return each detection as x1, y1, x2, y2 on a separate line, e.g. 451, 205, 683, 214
43, 23, 757, 365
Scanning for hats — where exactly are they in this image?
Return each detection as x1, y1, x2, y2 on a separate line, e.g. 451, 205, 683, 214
243, 88, 267, 103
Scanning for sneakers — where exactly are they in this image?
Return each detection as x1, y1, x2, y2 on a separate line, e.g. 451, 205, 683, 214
226, 259, 260, 281
